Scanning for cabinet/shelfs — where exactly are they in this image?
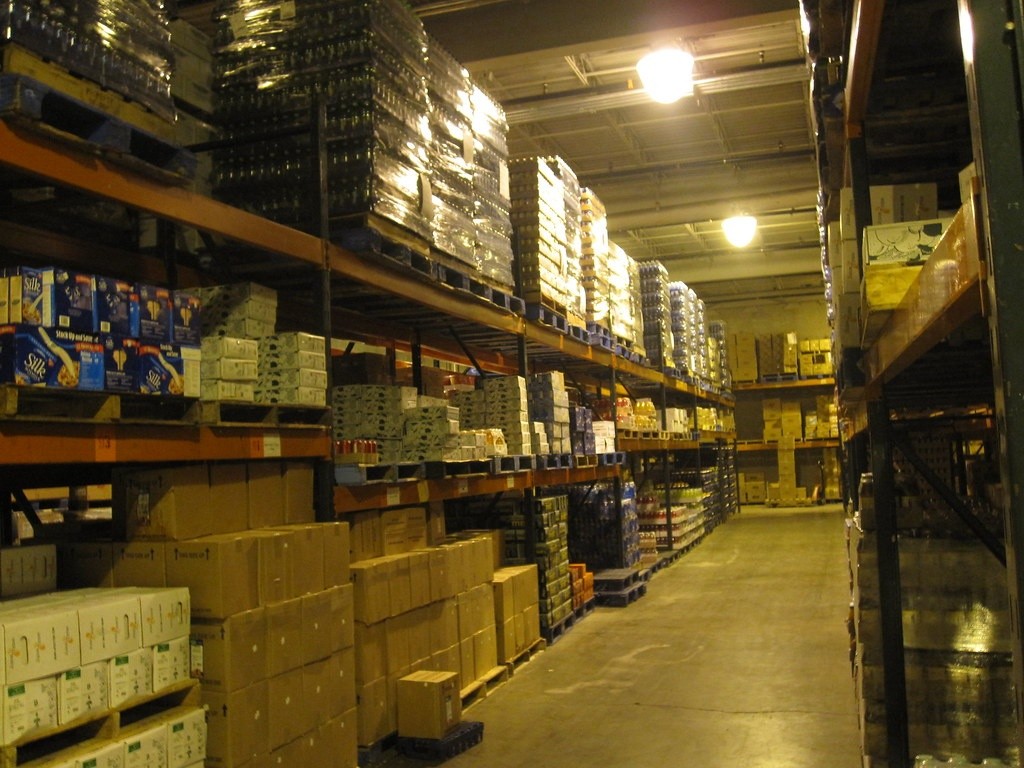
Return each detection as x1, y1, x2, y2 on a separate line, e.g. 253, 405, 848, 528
721, 381, 741, 523
641, 317, 705, 566
315, 105, 536, 743
510, 232, 625, 639
734, 379, 842, 507
0, 32, 353, 768
838, 0, 1023, 768
694, 372, 724, 536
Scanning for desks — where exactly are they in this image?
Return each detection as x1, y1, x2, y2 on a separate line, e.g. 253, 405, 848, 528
609, 295, 673, 588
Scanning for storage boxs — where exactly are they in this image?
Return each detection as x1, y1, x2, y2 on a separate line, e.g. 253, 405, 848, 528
482, 151, 740, 662
736, 327, 840, 506
798, 2, 1023, 767
0, 1, 500, 768
